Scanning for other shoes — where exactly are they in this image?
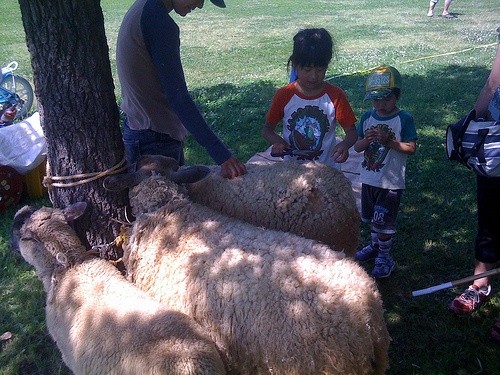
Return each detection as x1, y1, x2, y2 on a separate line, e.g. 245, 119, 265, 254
442, 13, 454, 18
427, 10, 434, 17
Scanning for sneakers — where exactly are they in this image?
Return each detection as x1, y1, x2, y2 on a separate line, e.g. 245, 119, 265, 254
491, 321, 500, 344
355, 244, 379, 261
448, 283, 494, 315
372, 255, 397, 277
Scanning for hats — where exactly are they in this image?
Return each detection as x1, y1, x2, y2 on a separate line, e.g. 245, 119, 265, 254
210, 0, 226, 8
445, 109, 477, 161
362, 65, 402, 101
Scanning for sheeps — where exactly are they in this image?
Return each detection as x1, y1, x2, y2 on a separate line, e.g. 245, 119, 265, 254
102, 155, 392, 375
9, 201, 230, 375
179, 158, 360, 260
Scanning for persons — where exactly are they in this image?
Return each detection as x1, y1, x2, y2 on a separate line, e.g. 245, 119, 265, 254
426, 0, 452, 17
449, 27, 500, 336
116, 0, 246, 178
354, 64, 417, 280
263, 28, 360, 172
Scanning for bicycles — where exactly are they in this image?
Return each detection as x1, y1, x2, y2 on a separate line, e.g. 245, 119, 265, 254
0, 61, 34, 121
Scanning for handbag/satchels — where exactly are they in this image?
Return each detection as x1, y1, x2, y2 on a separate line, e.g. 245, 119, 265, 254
458, 116, 500, 180
